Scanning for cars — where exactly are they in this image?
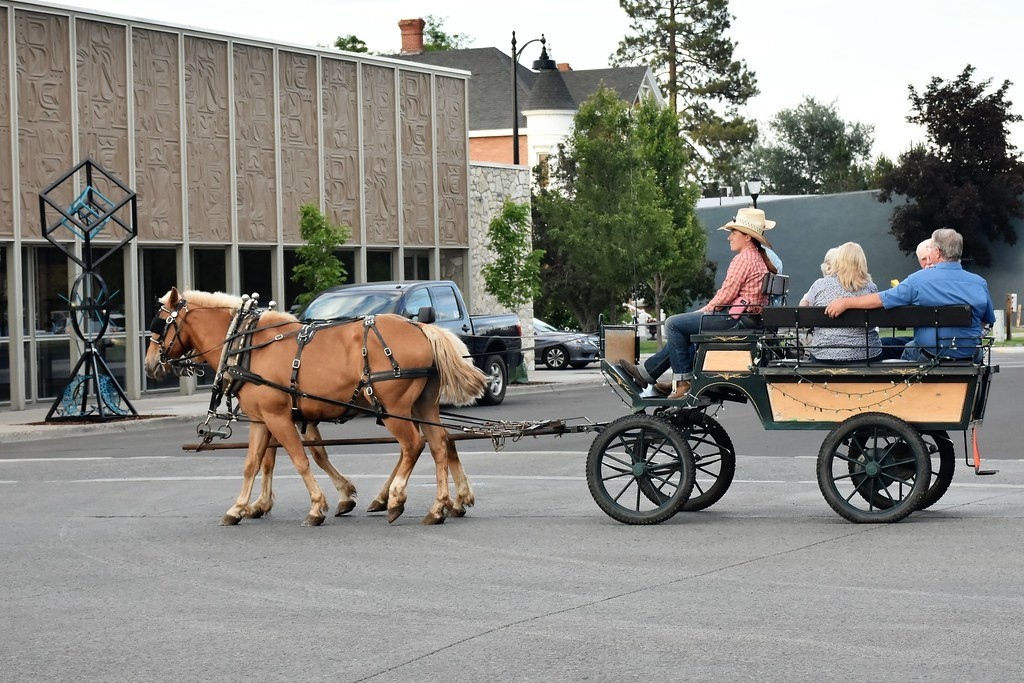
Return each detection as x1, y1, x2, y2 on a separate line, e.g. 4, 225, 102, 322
534, 317, 601, 370
622, 303, 652, 324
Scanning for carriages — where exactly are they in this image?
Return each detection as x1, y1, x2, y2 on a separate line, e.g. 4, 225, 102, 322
141, 208, 994, 525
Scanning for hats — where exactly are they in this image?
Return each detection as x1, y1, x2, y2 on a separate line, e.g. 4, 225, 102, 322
715, 208, 775, 230
724, 216, 772, 248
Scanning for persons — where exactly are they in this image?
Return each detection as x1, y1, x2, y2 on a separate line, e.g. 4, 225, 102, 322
878, 238, 937, 360
665, 215, 779, 400
824, 228, 997, 364
799, 245, 838, 308
807, 241, 884, 365
618, 207, 783, 396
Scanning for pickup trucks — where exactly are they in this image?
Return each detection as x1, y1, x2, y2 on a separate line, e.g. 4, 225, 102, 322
297, 280, 526, 405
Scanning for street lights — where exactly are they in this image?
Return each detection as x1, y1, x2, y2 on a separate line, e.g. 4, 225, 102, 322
749, 176, 762, 207
510, 30, 557, 165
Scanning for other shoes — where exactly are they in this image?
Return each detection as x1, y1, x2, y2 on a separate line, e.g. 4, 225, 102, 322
653, 382, 672, 395
619, 359, 648, 389
667, 381, 690, 397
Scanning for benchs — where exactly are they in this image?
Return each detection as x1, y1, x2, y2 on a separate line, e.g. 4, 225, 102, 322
690, 273, 790, 343
761, 305, 972, 366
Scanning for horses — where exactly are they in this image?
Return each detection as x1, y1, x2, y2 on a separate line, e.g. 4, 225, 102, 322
143, 286, 494, 528
